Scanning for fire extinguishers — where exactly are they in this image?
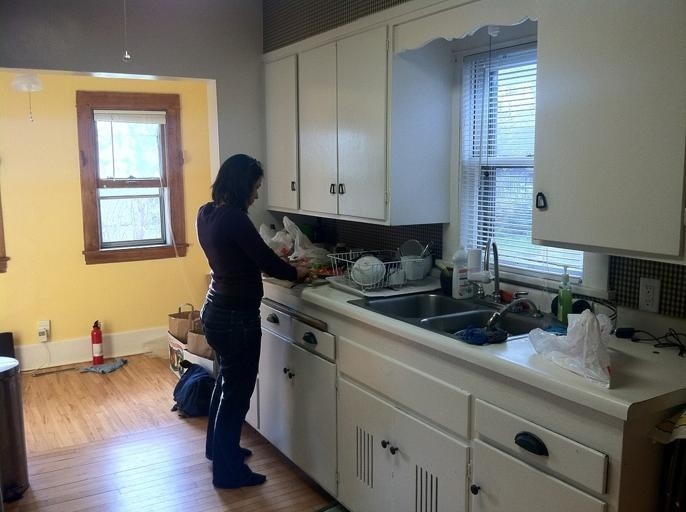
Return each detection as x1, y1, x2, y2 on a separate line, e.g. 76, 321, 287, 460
91, 327, 104, 366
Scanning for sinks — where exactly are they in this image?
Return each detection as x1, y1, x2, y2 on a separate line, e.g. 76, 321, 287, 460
347, 287, 503, 320
418, 309, 556, 345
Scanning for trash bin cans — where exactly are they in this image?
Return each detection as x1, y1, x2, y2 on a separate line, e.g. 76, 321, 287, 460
0, 356, 31, 500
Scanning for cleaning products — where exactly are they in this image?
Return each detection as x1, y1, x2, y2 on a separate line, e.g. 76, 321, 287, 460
451, 244, 474, 299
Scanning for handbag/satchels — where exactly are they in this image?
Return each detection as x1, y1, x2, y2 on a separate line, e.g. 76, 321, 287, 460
167, 303, 208, 344
173, 360, 219, 419
185, 318, 220, 359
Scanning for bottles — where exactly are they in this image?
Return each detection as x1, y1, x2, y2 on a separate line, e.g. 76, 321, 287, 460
335, 242, 346, 266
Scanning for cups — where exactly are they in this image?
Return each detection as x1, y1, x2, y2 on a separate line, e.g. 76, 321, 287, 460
390, 269, 405, 285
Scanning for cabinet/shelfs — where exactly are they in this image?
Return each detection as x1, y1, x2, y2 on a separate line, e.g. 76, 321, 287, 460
532, 0, 686, 267
337, 302, 470, 512
257, 274, 336, 501
263, 40, 300, 214
298, 8, 450, 227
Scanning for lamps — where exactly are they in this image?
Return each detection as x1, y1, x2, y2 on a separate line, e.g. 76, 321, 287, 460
119, 0, 134, 63
10, 69, 42, 124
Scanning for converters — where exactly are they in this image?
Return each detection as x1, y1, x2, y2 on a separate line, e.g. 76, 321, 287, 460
615, 327, 636, 339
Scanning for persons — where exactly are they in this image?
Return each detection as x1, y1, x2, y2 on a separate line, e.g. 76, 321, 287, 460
195, 152, 311, 490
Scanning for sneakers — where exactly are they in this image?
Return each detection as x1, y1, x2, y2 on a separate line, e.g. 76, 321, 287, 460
236, 447, 252, 459
213, 464, 267, 488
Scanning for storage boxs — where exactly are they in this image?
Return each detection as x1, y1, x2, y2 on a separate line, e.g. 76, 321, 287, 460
470, 334, 686, 512
183, 349, 221, 375
168, 331, 188, 379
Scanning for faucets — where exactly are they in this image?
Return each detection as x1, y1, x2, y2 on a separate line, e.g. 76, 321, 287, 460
486, 298, 545, 331
484, 239, 501, 303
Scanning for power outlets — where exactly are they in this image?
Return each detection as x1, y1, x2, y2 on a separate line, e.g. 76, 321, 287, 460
39, 320, 50, 337
639, 277, 661, 313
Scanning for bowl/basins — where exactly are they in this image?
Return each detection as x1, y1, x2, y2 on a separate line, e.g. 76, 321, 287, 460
351, 256, 386, 286
